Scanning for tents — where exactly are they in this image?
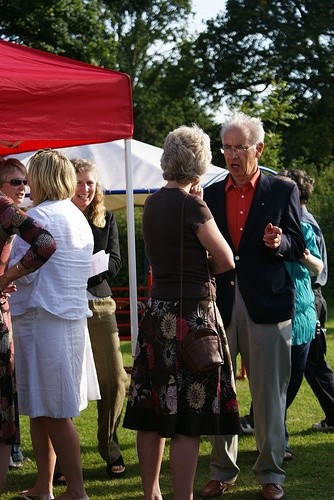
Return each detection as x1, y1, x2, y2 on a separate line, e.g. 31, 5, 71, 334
0, 40, 133, 156
2, 139, 232, 195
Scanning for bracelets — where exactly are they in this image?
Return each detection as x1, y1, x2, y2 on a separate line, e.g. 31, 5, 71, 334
16, 263, 23, 275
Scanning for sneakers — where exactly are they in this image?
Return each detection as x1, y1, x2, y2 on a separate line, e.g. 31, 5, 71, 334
239, 416, 255, 434
312, 420, 334, 430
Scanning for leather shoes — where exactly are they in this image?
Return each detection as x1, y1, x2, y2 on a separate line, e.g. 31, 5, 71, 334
262, 483, 284, 500
200, 480, 236, 497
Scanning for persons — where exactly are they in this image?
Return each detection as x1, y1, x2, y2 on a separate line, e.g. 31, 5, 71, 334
122, 126, 241, 500
204, 118, 306, 500
7, 147, 101, 500
0, 156, 334, 483
0, 193, 56, 491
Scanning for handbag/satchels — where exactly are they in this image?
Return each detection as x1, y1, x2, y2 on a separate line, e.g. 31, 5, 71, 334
181, 327, 226, 373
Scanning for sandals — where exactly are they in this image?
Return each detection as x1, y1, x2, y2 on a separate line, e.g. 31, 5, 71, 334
53, 473, 67, 487
107, 455, 126, 476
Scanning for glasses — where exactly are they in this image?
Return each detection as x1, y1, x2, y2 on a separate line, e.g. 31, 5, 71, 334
220, 145, 248, 155
4, 178, 27, 186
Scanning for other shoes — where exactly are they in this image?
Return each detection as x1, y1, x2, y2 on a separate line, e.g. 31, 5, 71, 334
282, 444, 294, 461
